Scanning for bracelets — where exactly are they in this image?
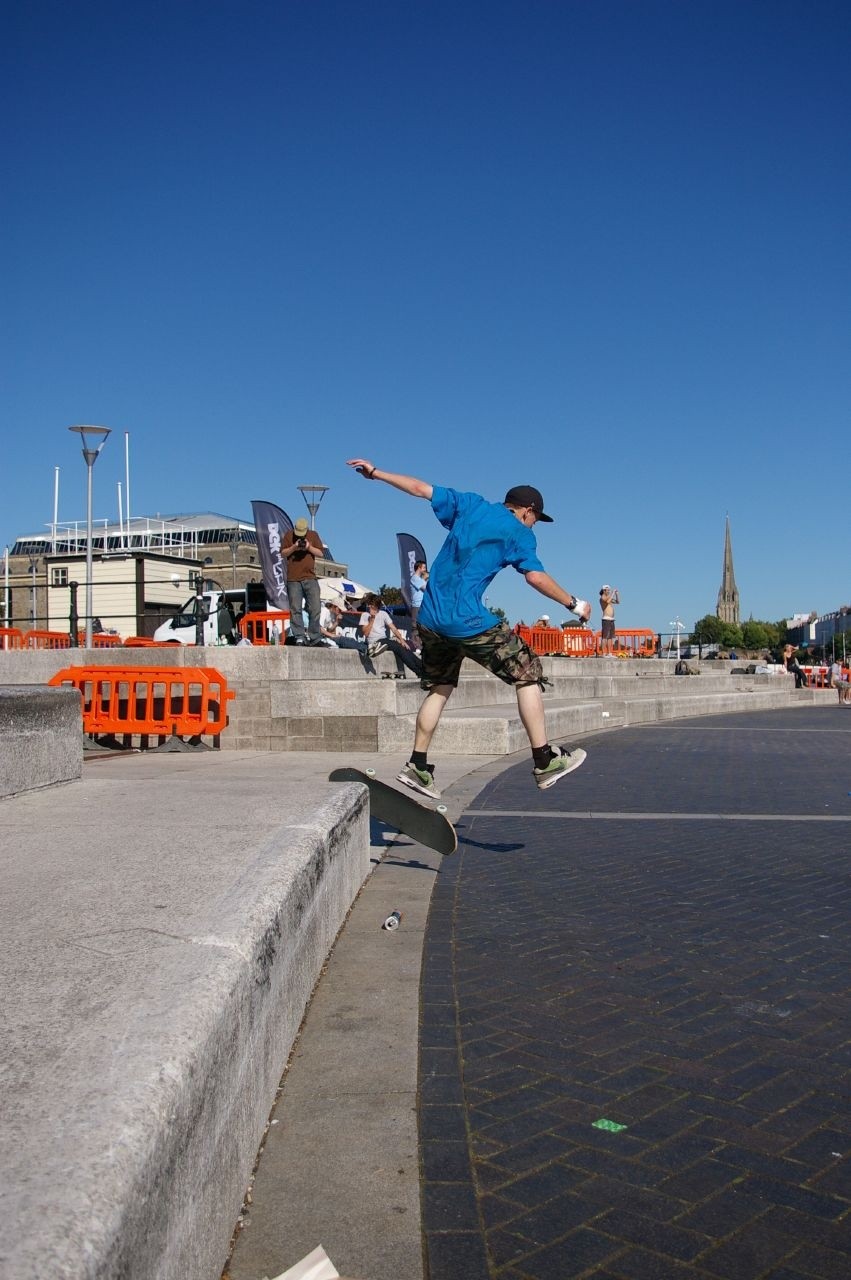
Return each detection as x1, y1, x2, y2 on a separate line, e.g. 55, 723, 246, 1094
565, 596, 577, 611
369, 468, 377, 480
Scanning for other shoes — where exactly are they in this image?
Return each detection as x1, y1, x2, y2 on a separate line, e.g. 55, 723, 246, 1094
315, 641, 331, 647
368, 642, 380, 653
602, 655, 610, 658
608, 654, 617, 658
370, 644, 389, 657
297, 639, 304, 645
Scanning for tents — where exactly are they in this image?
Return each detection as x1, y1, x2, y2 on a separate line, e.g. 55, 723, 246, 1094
315, 576, 384, 613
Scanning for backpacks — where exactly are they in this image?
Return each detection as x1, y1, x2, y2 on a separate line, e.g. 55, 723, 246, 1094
675, 660, 690, 674
731, 652, 736, 659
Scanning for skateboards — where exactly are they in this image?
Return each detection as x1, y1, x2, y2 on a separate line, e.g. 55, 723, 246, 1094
735, 687, 752, 692
636, 671, 665, 675
381, 671, 405, 680
329, 768, 458, 857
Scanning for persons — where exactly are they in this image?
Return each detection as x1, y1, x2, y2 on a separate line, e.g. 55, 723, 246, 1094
318, 594, 388, 660
730, 646, 737, 659
281, 519, 333, 648
827, 656, 851, 705
599, 585, 622, 660
345, 458, 587, 803
409, 559, 429, 651
783, 644, 809, 688
534, 615, 563, 658
358, 593, 434, 688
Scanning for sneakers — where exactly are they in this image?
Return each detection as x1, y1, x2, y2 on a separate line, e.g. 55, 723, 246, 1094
533, 744, 587, 789
396, 762, 441, 799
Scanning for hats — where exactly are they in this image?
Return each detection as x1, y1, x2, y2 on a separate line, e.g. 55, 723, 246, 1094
294, 519, 308, 536
507, 486, 554, 522
602, 584, 610, 589
331, 597, 347, 611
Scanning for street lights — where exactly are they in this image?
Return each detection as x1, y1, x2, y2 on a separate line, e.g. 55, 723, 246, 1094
66, 424, 113, 648
296, 485, 331, 531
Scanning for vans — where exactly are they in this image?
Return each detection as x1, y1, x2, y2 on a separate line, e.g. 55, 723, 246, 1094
154, 589, 291, 644
